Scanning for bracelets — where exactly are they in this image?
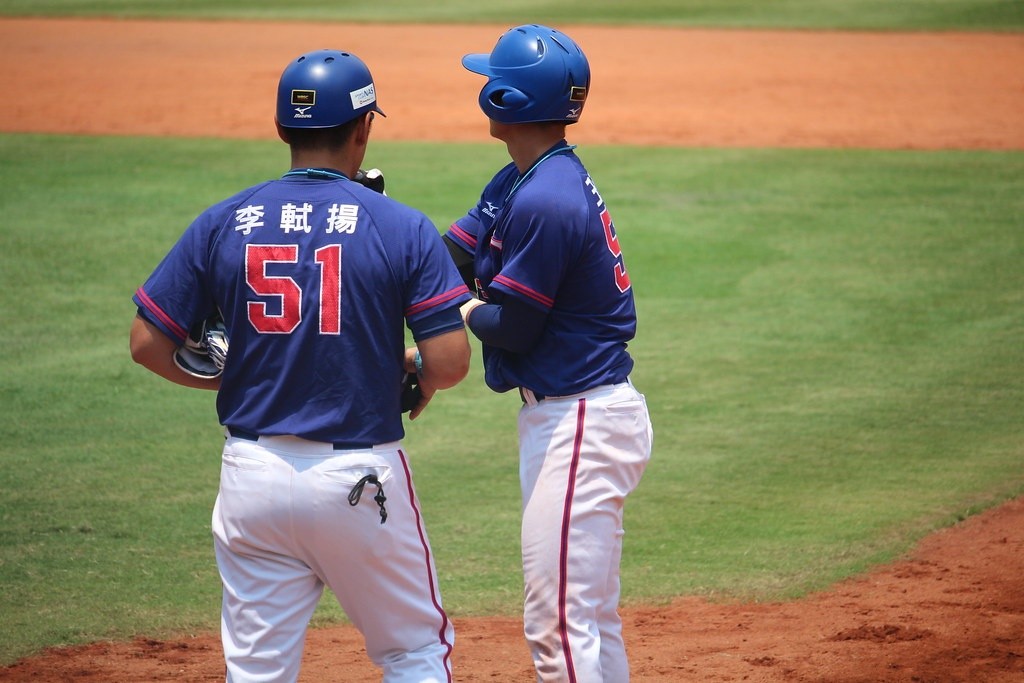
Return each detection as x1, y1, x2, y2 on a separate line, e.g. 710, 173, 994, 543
414, 349, 423, 378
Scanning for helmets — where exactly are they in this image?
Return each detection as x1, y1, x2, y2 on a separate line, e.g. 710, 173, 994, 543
277, 49, 387, 130
461, 23, 591, 124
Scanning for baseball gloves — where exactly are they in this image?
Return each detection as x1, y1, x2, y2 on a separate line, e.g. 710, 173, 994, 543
172, 304, 229, 381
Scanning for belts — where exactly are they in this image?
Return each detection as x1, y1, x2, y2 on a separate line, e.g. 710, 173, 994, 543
225, 426, 372, 450
520, 376, 627, 403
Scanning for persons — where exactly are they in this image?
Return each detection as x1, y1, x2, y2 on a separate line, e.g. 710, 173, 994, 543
442, 24, 654, 683
129, 49, 473, 683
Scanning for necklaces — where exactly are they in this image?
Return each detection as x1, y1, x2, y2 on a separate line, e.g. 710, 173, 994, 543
505, 145, 577, 201
281, 168, 351, 182
